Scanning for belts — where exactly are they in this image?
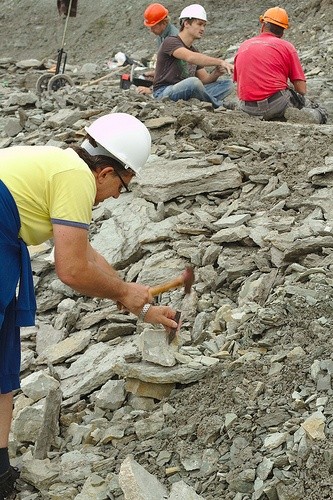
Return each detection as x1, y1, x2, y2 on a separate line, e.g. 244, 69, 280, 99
244, 91, 283, 107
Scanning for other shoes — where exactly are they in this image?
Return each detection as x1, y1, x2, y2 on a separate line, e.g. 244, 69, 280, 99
1, 465, 40, 498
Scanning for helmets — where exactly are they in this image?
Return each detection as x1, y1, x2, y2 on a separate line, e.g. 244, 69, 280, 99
179, 4, 210, 24
144, 3, 168, 27
84, 112, 152, 173
260, 6, 289, 29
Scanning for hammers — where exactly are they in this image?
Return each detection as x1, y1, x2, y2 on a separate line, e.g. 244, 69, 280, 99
149, 264, 196, 294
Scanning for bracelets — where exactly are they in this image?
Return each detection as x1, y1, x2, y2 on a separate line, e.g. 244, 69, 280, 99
138, 304, 151, 321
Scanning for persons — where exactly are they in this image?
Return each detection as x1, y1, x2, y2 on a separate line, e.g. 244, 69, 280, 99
233, 7, 306, 118
137, 2, 180, 94
0, 113, 182, 500
153, 3, 236, 108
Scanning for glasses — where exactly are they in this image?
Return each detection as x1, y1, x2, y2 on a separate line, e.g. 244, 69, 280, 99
110, 167, 133, 195
